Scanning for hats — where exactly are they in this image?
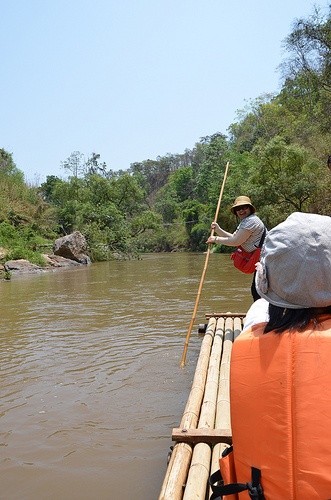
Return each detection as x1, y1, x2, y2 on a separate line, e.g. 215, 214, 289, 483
229, 195, 256, 215
255, 211, 331, 308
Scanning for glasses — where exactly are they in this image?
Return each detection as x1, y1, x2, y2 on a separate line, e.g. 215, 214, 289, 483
234, 205, 249, 211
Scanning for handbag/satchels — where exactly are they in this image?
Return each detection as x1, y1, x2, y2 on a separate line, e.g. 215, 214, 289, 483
229, 246, 261, 275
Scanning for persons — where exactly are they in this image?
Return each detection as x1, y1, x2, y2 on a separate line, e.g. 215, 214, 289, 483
205, 195, 267, 302
228, 212, 331, 500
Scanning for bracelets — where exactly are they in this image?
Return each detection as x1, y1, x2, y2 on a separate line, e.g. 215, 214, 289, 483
214, 236, 218, 244
